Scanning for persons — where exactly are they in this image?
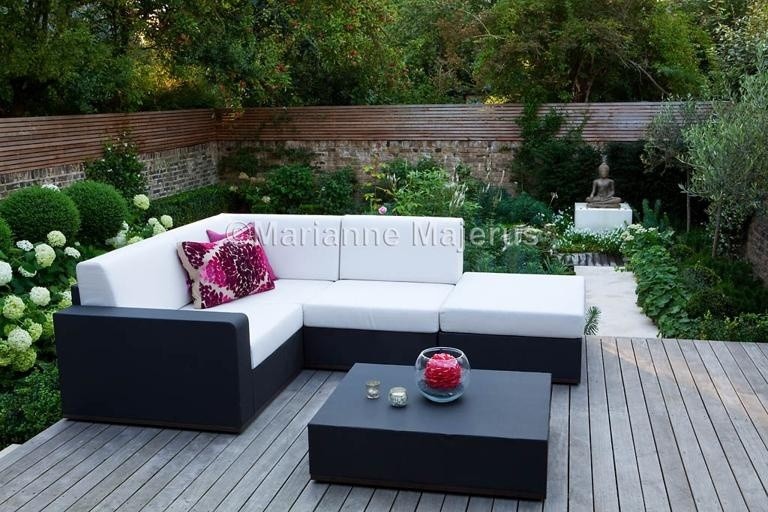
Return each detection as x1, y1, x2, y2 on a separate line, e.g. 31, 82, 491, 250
586, 154, 622, 207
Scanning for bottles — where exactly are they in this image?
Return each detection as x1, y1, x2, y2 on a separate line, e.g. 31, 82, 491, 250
365, 380, 380, 399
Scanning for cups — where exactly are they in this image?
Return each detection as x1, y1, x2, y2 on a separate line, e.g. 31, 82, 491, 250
388, 387, 409, 408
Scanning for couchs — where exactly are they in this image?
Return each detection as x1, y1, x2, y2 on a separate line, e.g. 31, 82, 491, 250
440, 272, 584, 385
52, 213, 342, 434
304, 215, 464, 371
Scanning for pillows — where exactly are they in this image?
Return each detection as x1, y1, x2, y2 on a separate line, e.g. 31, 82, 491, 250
206, 222, 279, 282
177, 228, 275, 309
